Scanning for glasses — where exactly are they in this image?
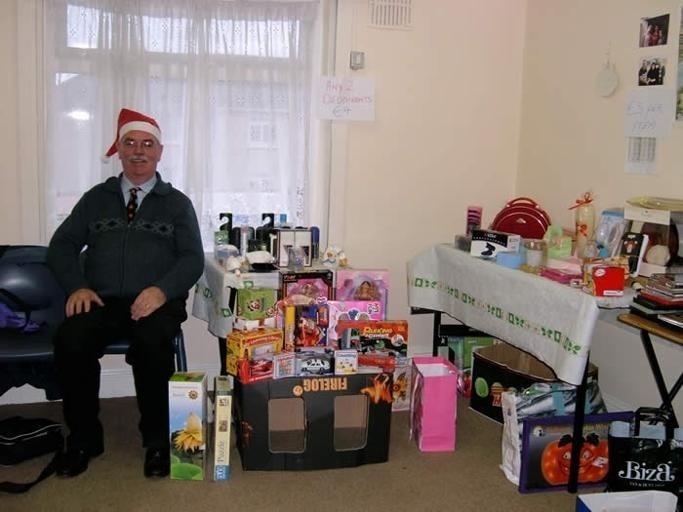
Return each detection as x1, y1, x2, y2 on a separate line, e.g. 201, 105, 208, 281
116, 138, 155, 155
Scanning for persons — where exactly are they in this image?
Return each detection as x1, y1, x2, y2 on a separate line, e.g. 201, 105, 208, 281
43, 108, 206, 478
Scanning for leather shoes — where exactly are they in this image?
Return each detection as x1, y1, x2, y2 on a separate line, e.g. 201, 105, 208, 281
58, 429, 105, 477
144, 445, 169, 480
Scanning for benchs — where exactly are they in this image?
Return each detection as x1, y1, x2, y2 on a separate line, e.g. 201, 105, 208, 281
0, 245, 186, 374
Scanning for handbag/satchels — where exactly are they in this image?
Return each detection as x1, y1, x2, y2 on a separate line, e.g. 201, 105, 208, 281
606, 405, 683, 493
409, 354, 459, 454
492, 195, 551, 240
0, 414, 63, 494
501, 380, 606, 495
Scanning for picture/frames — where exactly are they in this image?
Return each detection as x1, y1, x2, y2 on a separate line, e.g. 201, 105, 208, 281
518, 410, 634, 493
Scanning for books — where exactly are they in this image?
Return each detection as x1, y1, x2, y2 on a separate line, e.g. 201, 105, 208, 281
629, 270, 683, 336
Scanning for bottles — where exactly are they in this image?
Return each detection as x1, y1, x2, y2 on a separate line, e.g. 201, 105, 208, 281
310, 226, 320, 262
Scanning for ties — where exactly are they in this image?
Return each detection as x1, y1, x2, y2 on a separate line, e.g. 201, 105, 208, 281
126, 187, 137, 221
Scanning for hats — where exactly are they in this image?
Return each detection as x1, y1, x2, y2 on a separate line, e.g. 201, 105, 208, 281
101, 106, 163, 161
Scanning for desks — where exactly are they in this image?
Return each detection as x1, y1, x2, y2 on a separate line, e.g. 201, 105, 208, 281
616, 313, 683, 434
405, 242, 638, 494
191, 252, 337, 373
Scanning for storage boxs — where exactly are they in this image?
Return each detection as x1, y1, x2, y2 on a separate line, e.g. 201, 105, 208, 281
235, 371, 394, 472
468, 341, 599, 425
168, 373, 206, 482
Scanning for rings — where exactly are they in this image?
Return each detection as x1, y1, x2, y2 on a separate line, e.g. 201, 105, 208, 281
144, 303, 149, 308
